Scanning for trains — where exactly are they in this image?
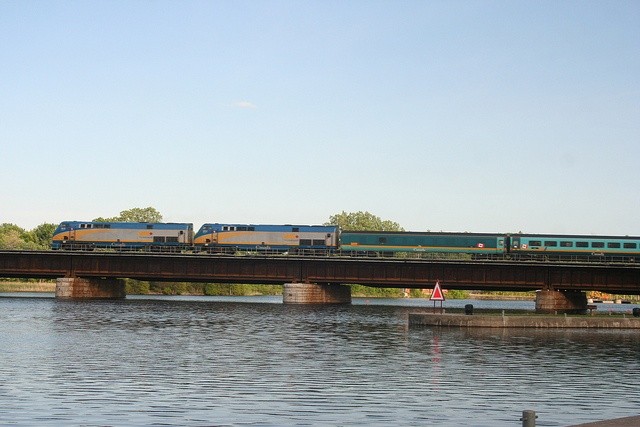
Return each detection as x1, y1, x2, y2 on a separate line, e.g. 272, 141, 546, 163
48, 220, 640, 263
